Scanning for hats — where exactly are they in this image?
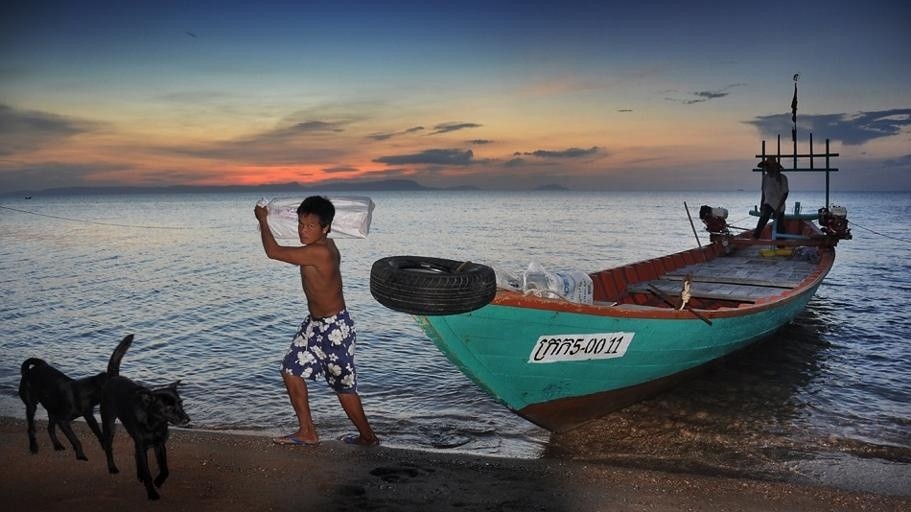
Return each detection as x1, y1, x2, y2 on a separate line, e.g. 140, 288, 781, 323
759, 156, 785, 171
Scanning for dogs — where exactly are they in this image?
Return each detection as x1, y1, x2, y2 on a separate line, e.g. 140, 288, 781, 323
19, 358, 107, 461
99, 335, 190, 502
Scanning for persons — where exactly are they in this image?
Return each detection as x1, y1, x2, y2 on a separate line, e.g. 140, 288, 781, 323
751, 157, 788, 249
253, 194, 380, 447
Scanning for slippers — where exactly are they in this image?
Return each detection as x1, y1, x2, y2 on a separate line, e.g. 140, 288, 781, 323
344, 434, 380, 446
272, 435, 321, 448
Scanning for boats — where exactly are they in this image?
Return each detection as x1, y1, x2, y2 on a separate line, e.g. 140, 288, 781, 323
369, 73, 853, 433
